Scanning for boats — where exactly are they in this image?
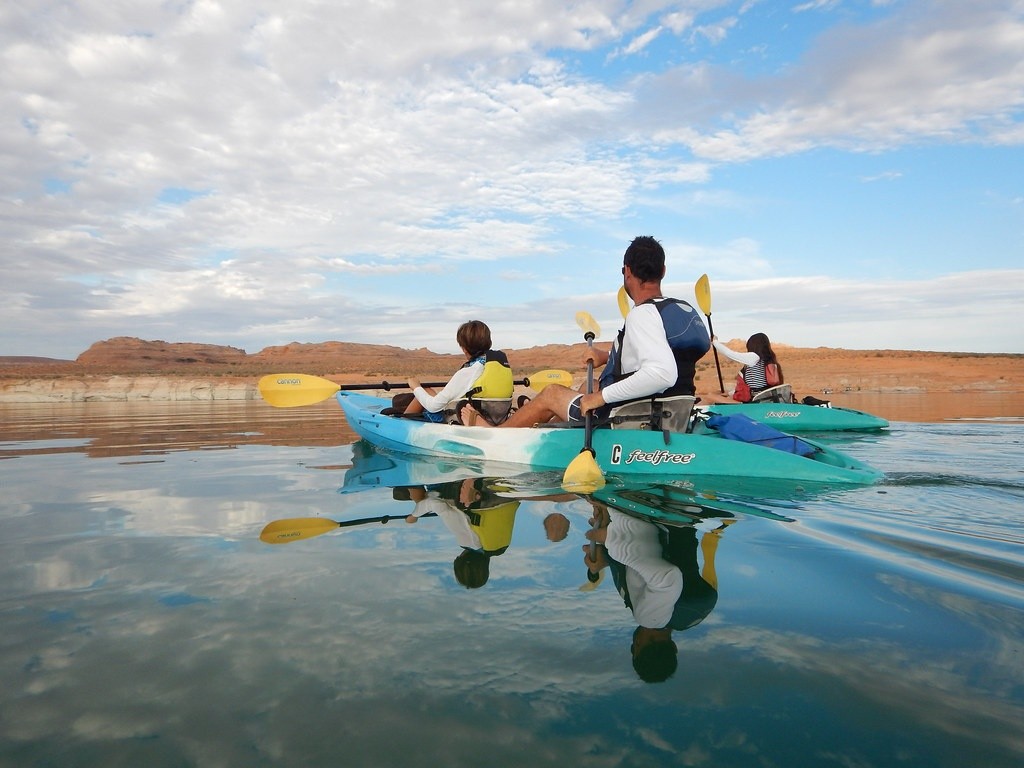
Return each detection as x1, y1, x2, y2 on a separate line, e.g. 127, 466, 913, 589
692, 403, 889, 431
336, 391, 885, 485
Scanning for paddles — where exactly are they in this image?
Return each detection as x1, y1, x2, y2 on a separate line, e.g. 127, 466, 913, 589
260, 512, 438, 545
258, 369, 573, 409
617, 284, 629, 319
694, 273, 725, 394
563, 312, 605, 484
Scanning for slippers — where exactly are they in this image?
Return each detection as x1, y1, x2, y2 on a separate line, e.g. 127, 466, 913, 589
517, 394, 533, 409
454, 399, 487, 427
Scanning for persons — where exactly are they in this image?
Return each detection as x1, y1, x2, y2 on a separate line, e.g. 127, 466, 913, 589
519, 493, 717, 685
403, 485, 520, 589
456, 235, 710, 427
400, 318, 512, 426
697, 332, 784, 405
